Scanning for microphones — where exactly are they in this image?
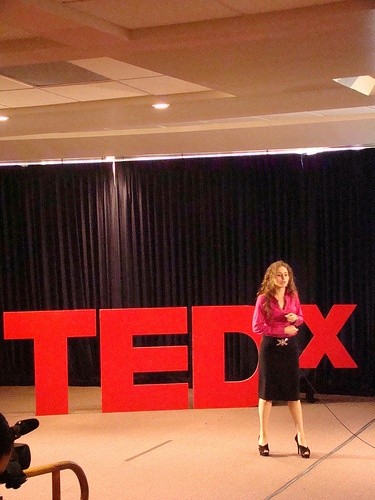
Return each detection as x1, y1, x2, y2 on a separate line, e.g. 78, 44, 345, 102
16, 419, 40, 436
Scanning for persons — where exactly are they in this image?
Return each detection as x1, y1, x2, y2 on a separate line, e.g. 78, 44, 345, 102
253, 261, 310, 458
0, 412, 14, 476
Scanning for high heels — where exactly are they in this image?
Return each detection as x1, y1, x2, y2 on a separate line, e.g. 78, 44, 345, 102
258, 435, 269, 456
295, 434, 310, 458
